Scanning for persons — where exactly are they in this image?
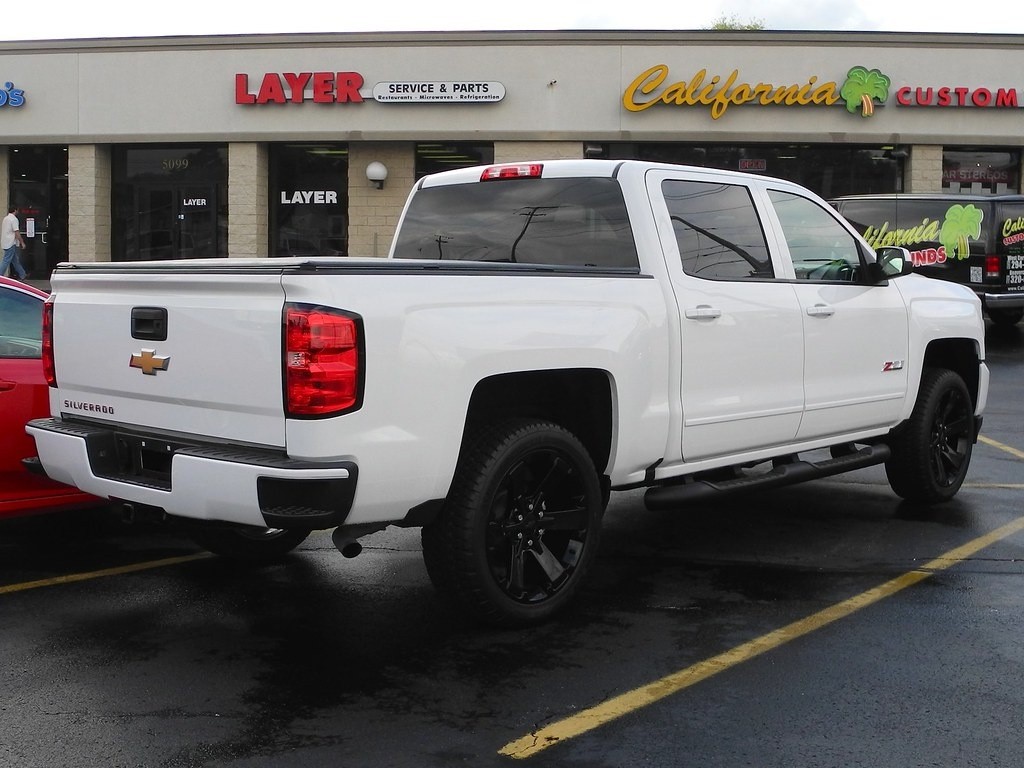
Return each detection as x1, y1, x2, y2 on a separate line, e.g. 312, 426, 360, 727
0, 204, 32, 280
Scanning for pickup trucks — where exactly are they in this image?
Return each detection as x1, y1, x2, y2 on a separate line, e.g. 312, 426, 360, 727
26, 159, 989, 630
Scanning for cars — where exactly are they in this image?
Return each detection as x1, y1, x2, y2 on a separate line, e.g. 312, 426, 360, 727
1, 275, 118, 518
822, 192, 1024, 327
128, 229, 196, 261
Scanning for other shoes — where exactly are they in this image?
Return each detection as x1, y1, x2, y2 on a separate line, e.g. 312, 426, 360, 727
20, 273, 30, 282
